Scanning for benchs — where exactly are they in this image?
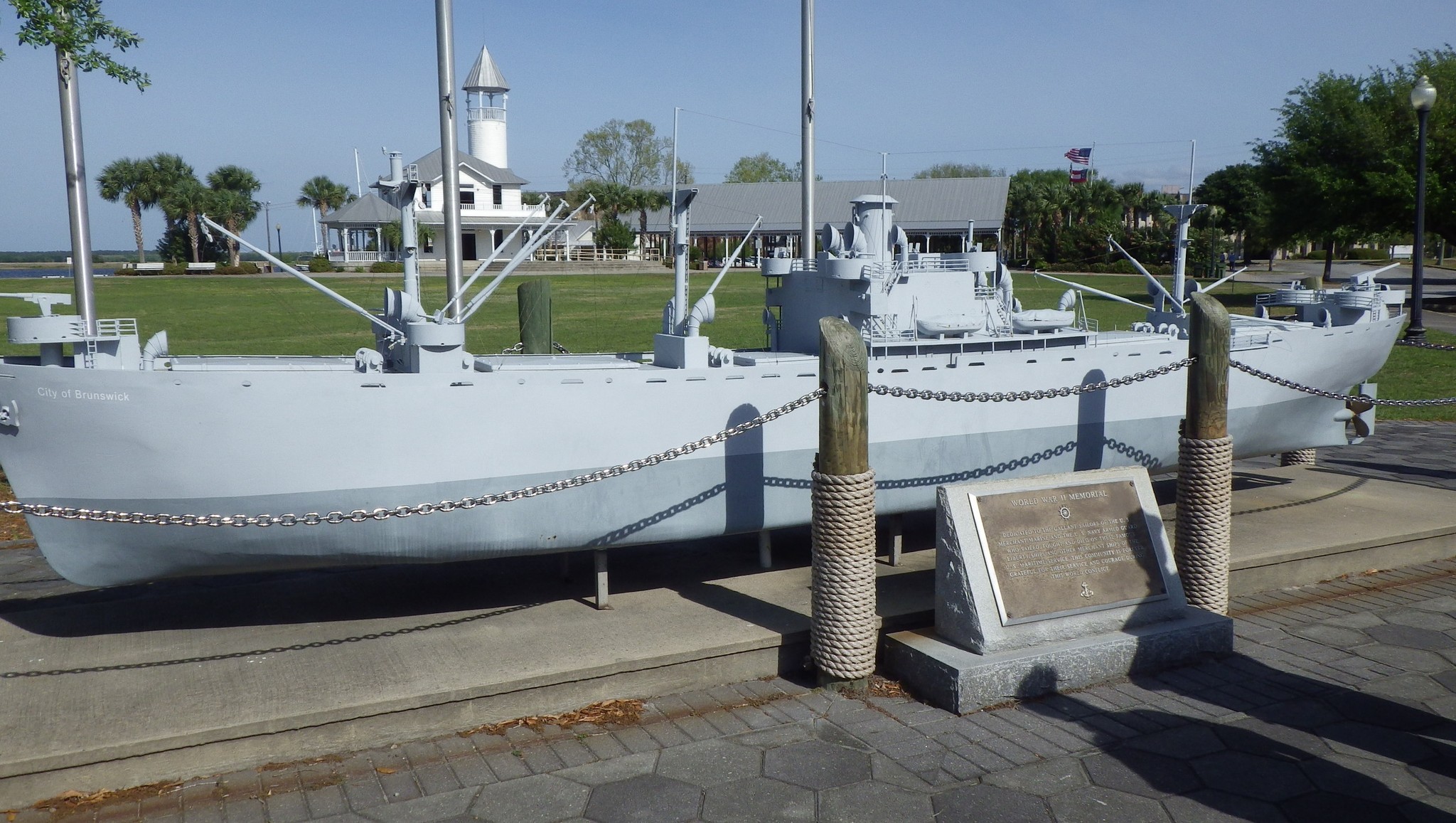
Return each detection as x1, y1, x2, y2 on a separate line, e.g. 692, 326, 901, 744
186, 263, 215, 275
255, 262, 264, 274
133, 263, 164, 275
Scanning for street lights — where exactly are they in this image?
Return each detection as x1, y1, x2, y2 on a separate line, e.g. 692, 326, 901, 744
259, 200, 272, 261
1399, 73, 1438, 343
273, 222, 284, 272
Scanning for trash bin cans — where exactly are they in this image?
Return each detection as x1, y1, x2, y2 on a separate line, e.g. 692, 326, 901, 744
699, 261, 708, 270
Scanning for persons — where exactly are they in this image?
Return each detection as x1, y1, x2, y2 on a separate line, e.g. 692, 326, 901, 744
990, 244, 998, 251
944, 244, 951, 253
1228, 251, 1235, 271
1220, 252, 1225, 263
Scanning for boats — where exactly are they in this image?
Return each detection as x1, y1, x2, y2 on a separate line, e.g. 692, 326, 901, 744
1, 0, 1411, 597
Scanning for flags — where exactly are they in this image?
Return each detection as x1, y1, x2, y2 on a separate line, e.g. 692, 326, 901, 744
1070, 169, 1087, 182
1064, 148, 1092, 166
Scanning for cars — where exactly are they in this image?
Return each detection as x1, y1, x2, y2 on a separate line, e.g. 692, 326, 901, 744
707, 256, 763, 267
